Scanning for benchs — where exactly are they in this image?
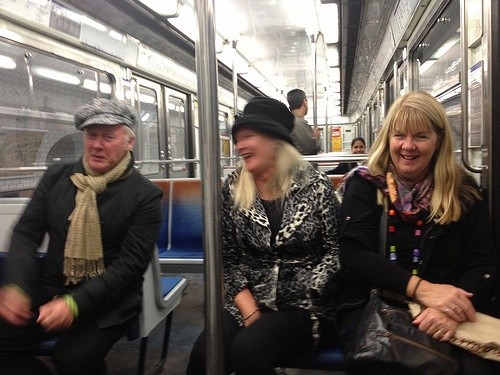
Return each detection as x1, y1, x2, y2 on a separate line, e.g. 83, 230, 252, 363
148, 178, 224, 274
0, 198, 186, 375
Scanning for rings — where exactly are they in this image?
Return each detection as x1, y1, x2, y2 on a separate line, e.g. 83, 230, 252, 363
437, 329, 445, 338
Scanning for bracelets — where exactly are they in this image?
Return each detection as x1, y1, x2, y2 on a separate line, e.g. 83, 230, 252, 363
243, 307, 259, 321
413, 278, 424, 298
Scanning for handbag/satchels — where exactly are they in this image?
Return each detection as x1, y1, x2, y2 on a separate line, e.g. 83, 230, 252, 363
336, 281, 492, 371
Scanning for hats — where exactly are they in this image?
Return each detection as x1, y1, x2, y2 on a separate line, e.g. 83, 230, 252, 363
231, 97, 295, 145
73, 99, 136, 128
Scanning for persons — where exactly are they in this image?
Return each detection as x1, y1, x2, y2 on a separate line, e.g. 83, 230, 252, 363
342, 91, 500, 375
0, 98, 164, 375
34, 106, 76, 168
186, 96, 341, 375
323, 137, 368, 174
287, 89, 321, 155
